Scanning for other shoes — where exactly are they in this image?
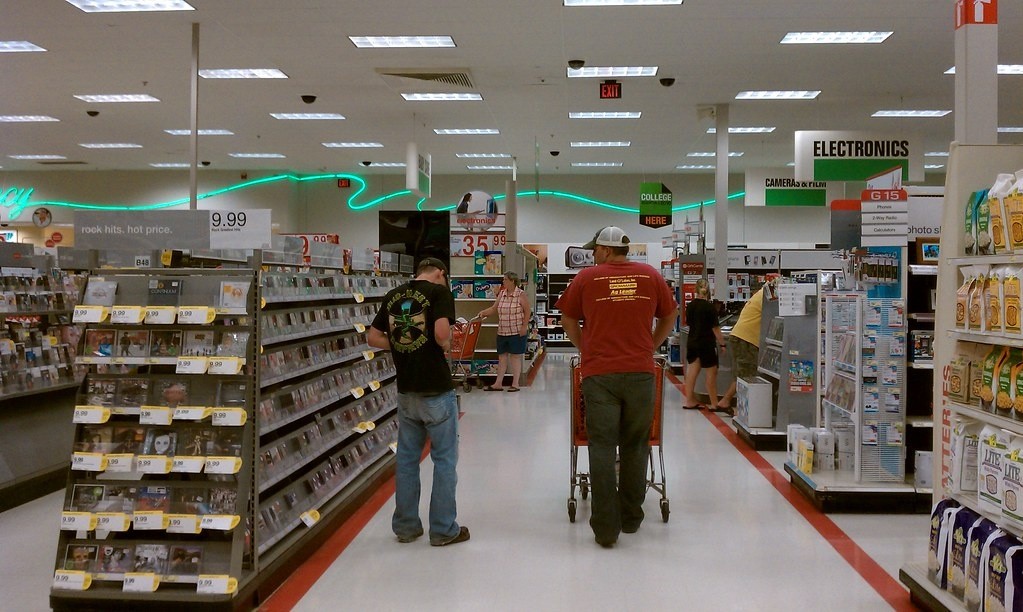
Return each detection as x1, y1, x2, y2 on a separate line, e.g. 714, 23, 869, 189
623, 506, 643, 533
447, 526, 470, 543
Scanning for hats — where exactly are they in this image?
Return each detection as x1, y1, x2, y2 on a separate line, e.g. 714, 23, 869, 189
583, 224, 631, 249
418, 257, 450, 288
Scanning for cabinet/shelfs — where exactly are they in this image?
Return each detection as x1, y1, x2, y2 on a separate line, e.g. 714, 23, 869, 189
446, 248, 580, 391
897, 206, 1023, 612
905, 232, 937, 512
49, 265, 400, 612
0, 264, 84, 404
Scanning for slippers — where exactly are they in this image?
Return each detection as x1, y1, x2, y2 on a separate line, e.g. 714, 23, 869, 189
683, 402, 705, 408
709, 406, 725, 412
716, 405, 734, 417
508, 386, 520, 392
484, 384, 503, 391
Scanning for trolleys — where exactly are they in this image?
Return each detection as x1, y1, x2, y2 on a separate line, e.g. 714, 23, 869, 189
567, 354, 671, 524
445, 315, 484, 393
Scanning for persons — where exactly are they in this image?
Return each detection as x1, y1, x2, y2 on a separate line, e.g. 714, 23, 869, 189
553, 226, 679, 545
367, 257, 470, 547
716, 280, 764, 416
478, 271, 531, 392
682, 279, 726, 412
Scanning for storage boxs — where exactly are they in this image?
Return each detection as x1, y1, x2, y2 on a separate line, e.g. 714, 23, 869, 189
784, 423, 855, 476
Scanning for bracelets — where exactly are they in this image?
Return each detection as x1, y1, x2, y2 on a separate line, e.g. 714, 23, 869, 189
720, 345, 726, 347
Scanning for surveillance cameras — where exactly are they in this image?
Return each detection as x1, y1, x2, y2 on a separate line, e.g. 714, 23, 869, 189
301, 96, 316, 103
568, 60, 585, 70
363, 162, 371, 166
550, 151, 559, 156
659, 78, 675, 86
87, 111, 99, 116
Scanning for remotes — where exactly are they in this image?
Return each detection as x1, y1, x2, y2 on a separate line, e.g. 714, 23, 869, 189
201, 162, 210, 165
